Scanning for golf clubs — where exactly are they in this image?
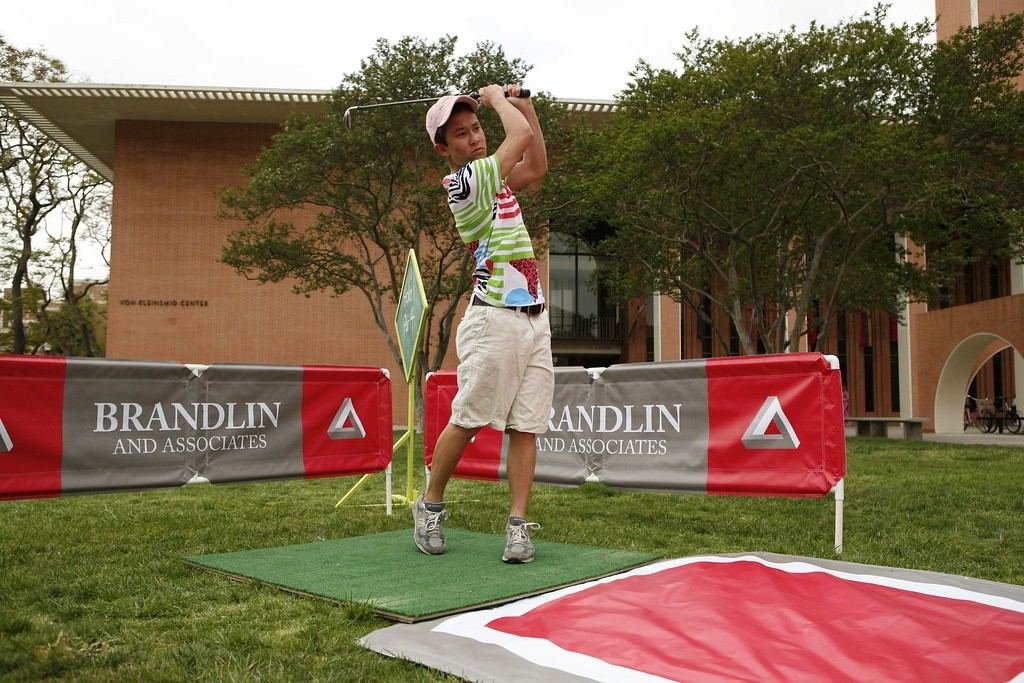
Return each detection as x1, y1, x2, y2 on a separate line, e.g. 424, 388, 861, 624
342, 88, 531, 132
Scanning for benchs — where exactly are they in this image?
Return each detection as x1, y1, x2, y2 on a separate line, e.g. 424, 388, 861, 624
975, 415, 1024, 433
844, 418, 929, 442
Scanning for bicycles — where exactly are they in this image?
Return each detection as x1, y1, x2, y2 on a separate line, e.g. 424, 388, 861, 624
982, 393, 1021, 434
964, 394, 993, 434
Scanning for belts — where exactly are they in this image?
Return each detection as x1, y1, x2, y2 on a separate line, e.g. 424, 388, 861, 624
472, 294, 545, 316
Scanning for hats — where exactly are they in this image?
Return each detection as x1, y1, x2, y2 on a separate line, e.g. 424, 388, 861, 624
425, 95, 479, 144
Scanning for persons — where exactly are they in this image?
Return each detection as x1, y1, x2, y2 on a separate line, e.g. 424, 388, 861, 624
843, 383, 852, 426
412, 84, 555, 564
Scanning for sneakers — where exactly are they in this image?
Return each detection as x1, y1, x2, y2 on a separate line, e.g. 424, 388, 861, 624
412, 490, 447, 555
502, 515, 541, 564
